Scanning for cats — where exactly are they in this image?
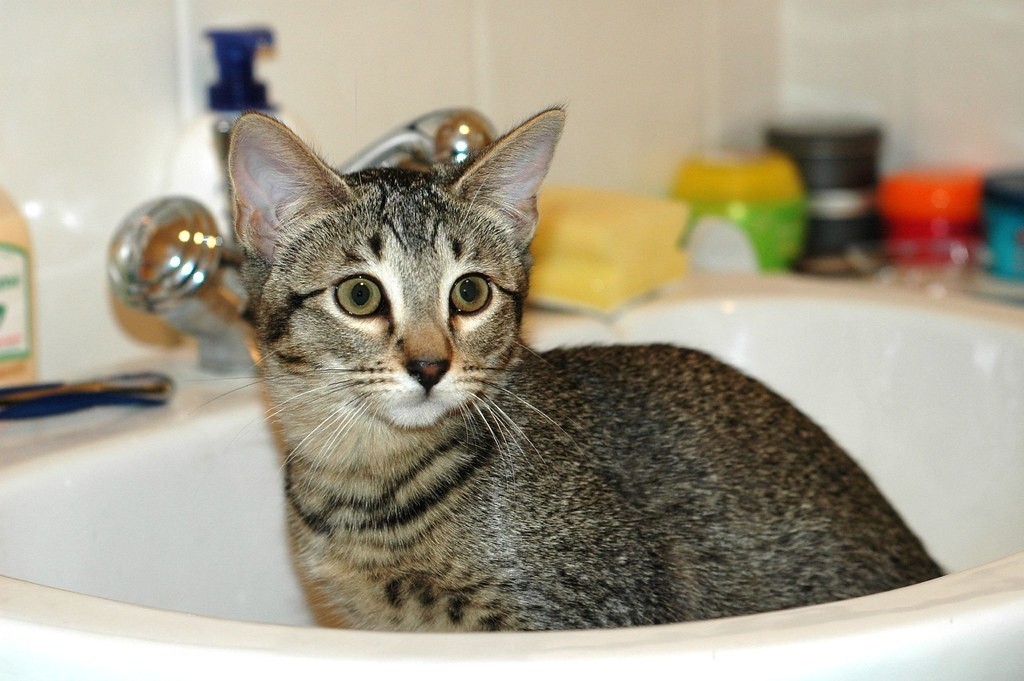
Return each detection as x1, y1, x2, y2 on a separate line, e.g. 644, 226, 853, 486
227, 105, 948, 632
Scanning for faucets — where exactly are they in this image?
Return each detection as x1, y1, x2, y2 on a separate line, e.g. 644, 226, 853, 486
339, 101, 499, 180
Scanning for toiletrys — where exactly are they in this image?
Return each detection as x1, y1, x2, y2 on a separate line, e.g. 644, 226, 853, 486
176, 23, 308, 378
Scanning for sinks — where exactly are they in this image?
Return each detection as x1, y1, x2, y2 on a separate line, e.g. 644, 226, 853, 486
0, 265, 1024, 679
